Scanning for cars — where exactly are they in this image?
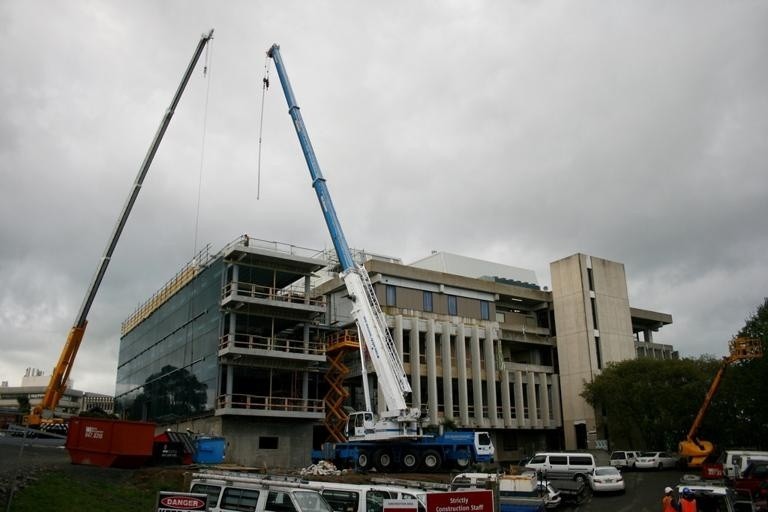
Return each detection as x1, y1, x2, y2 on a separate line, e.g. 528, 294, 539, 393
584, 464, 625, 491
634, 451, 679, 471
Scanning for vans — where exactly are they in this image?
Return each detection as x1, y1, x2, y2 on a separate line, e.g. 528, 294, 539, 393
525, 451, 596, 484
672, 449, 767, 512
610, 450, 642, 471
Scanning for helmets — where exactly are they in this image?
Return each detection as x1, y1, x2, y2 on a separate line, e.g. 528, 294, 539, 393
664, 486, 690, 495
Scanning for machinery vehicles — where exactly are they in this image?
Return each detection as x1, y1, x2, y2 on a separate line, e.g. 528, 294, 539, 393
676, 336, 762, 470
22, 23, 217, 433
256, 44, 495, 470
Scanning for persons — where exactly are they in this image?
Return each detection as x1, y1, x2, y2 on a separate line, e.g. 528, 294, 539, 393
662, 486, 682, 511
678, 487, 697, 511
